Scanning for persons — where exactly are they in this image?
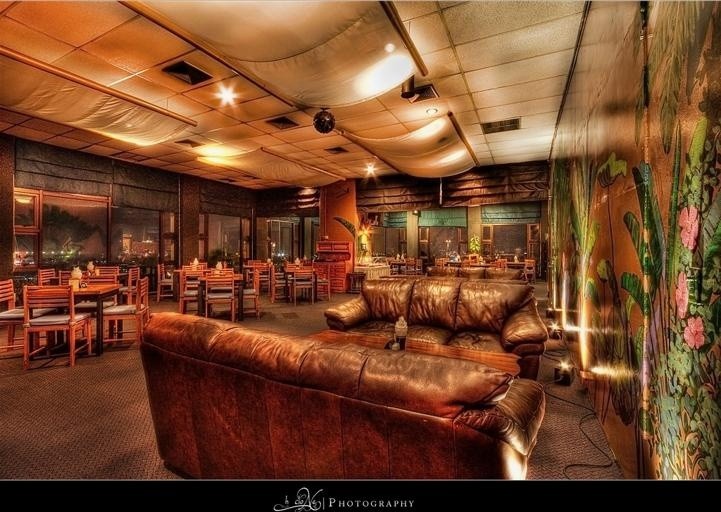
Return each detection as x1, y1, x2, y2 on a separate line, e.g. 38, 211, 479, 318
419, 250, 428, 276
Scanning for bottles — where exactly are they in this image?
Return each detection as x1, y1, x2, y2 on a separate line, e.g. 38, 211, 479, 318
70, 261, 100, 287
394, 315, 408, 352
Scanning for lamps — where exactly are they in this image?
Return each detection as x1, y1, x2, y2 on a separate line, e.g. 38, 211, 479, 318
554, 363, 576, 386
547, 324, 562, 339
544, 306, 555, 319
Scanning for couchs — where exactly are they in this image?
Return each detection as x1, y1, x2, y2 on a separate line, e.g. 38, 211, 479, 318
136, 308, 548, 483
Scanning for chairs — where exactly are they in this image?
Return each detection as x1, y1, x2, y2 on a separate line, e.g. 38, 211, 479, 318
0, 251, 548, 378
